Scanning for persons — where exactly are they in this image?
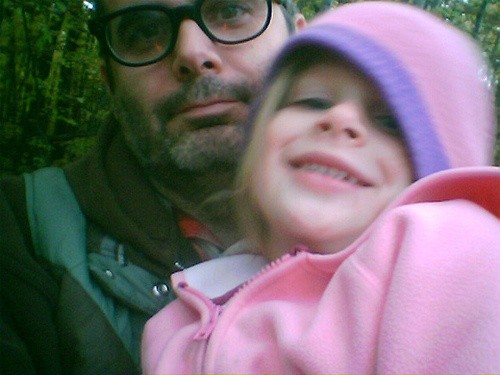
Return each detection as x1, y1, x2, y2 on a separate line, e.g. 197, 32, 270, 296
138, 0, 500, 375
0, 0, 305, 375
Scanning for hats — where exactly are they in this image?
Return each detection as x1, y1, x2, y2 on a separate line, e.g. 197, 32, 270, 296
232, 0, 497, 182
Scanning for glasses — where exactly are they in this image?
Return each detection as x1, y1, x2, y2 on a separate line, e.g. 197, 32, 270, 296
86, 0, 283, 67
